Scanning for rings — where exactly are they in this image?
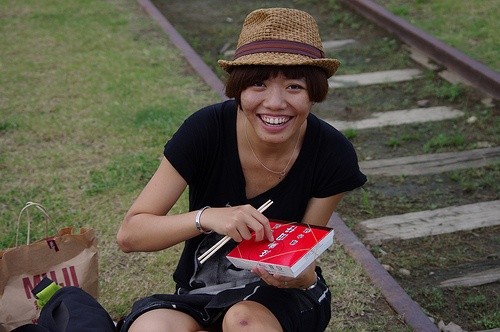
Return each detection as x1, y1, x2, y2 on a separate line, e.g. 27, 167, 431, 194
284, 281, 288, 285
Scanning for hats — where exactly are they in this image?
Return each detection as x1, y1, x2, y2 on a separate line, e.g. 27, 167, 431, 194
218, 8, 341, 79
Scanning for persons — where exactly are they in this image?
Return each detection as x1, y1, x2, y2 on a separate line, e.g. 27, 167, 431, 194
115, 6, 370, 332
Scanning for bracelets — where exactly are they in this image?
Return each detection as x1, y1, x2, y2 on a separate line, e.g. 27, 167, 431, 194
195, 206, 213, 234
301, 271, 317, 290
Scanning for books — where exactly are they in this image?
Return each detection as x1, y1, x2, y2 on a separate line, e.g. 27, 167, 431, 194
226, 218, 335, 279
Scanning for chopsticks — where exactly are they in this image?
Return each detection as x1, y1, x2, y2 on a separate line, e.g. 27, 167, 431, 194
197, 200, 274, 264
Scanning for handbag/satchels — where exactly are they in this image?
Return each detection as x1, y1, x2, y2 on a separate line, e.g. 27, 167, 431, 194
0, 202, 115, 332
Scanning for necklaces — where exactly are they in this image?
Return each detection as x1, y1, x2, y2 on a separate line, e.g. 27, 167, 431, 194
244, 114, 302, 177
